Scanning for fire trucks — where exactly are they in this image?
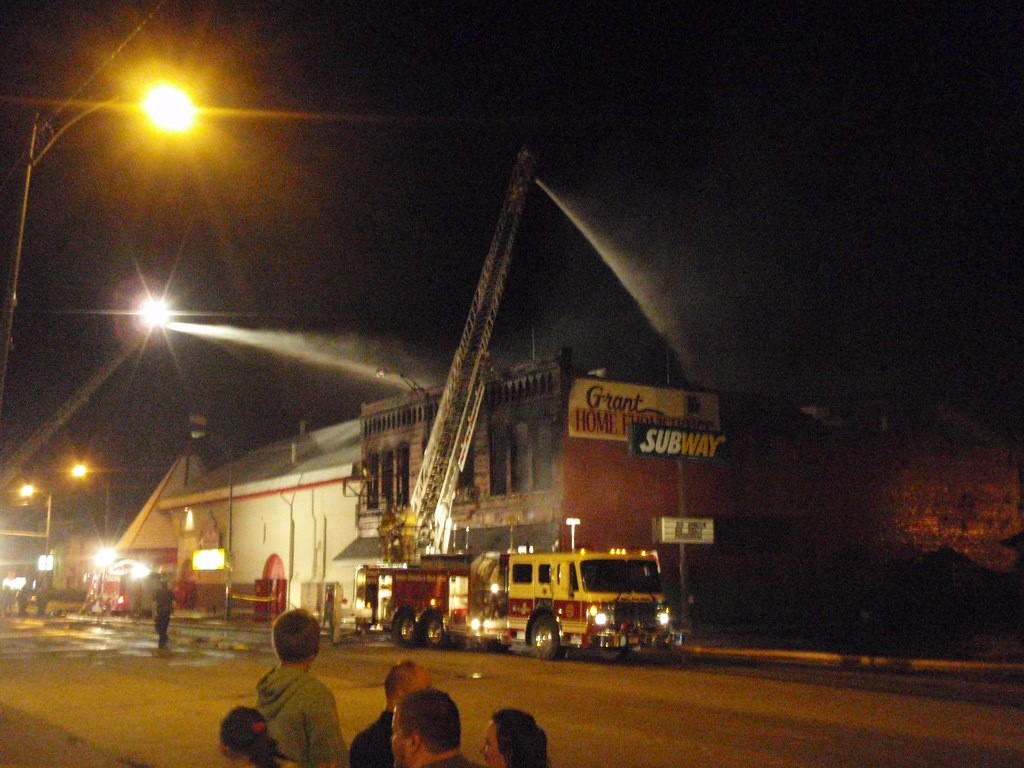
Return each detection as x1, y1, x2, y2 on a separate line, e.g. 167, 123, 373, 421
355, 140, 677, 658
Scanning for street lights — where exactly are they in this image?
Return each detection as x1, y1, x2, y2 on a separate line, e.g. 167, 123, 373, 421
19, 485, 54, 558
70, 462, 112, 549
0, 75, 201, 396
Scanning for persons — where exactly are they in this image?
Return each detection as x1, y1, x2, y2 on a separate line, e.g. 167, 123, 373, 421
253, 608, 347, 768
482, 707, 547, 768
16, 588, 31, 616
392, 688, 485, 768
2, 587, 13, 616
351, 660, 432, 768
35, 586, 51, 617
220, 706, 300, 768
152, 581, 176, 649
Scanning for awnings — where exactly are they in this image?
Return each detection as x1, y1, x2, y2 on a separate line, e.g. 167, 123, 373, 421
333, 535, 414, 560
439, 523, 560, 556
